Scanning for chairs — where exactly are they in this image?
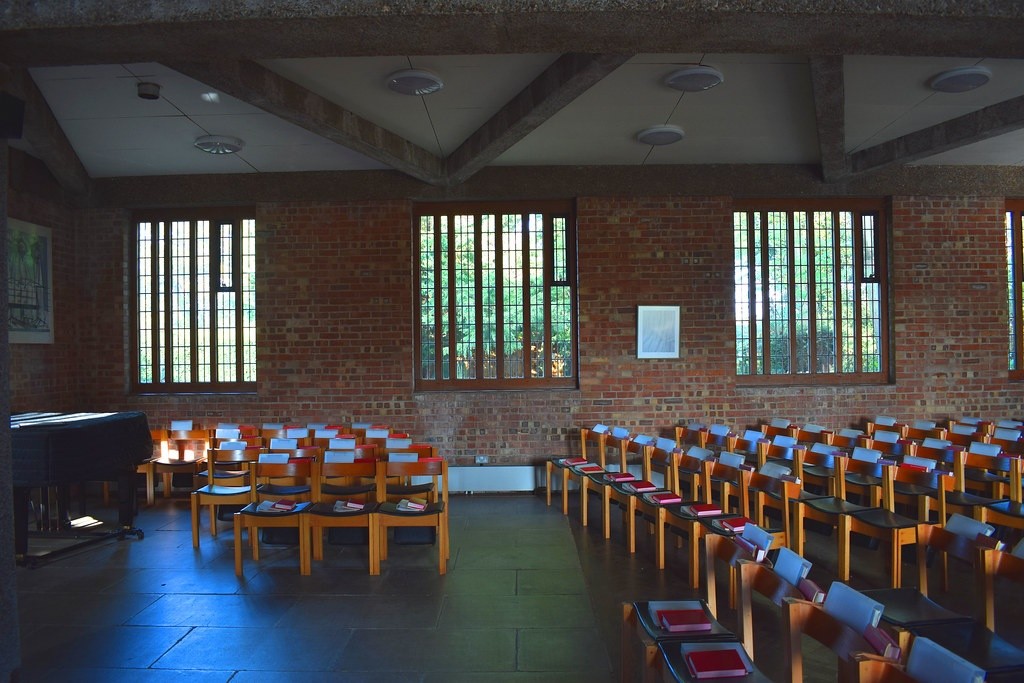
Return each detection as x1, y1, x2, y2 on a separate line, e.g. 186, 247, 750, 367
544, 415, 1023, 683
104, 422, 449, 578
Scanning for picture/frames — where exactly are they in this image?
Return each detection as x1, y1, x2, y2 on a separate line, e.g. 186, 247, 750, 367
636, 303, 681, 360
7, 217, 55, 344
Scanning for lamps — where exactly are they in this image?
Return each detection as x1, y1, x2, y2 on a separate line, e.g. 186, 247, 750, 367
193, 135, 242, 155
474, 455, 488, 463
664, 64, 723, 93
931, 68, 993, 94
384, 68, 444, 95
637, 125, 685, 145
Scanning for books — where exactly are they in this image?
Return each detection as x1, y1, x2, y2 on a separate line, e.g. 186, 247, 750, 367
569, 463, 605, 473
681, 642, 754, 680
603, 473, 635, 482
799, 577, 826, 604
622, 481, 658, 492
681, 504, 723, 517
643, 491, 682, 504
648, 601, 712, 632
863, 623, 901, 659
396, 497, 428, 512
333, 497, 365, 512
256, 499, 298, 513
735, 533, 764, 563
559, 457, 587, 466
589, 415, 1024, 551
712, 517, 757, 531
